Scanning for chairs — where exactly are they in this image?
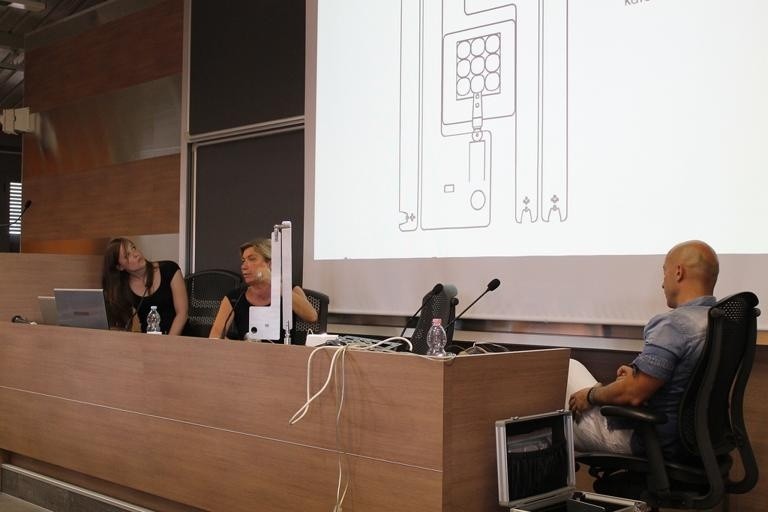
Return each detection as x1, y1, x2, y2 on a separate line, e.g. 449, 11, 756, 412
574, 291, 758, 511
184, 268, 329, 345
407, 286, 458, 355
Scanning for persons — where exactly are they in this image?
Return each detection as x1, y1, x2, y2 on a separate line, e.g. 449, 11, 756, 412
101, 238, 208, 337
208, 238, 319, 344
560, 239, 723, 454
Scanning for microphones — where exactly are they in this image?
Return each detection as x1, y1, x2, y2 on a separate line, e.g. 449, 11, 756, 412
397, 284, 443, 344
0, 195, 32, 234
124, 275, 154, 331
441, 276, 502, 344
220, 279, 249, 339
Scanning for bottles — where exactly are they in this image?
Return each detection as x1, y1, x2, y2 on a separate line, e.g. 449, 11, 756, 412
426, 318, 448, 358
146, 306, 161, 334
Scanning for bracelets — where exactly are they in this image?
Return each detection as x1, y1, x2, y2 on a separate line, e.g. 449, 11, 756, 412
587, 387, 595, 406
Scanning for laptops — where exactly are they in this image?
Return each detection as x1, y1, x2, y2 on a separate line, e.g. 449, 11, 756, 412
53, 287, 111, 328
36, 294, 56, 326
